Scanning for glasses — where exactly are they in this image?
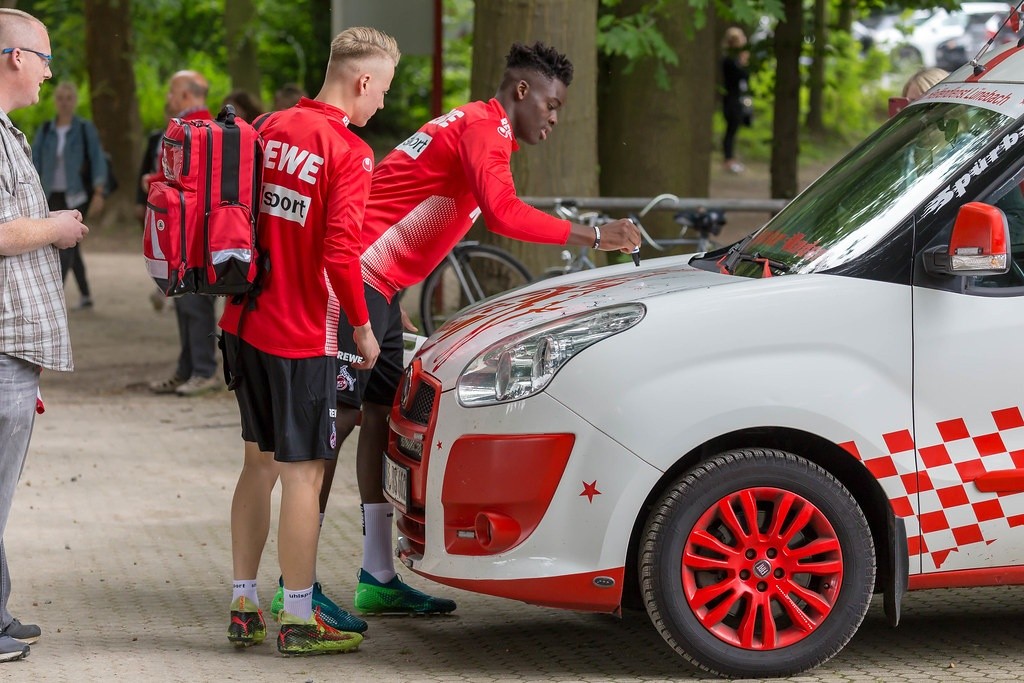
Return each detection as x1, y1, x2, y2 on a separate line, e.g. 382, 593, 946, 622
3, 45, 55, 68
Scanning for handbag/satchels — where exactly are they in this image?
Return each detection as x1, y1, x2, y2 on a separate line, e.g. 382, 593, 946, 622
143, 104, 286, 301
79, 121, 118, 201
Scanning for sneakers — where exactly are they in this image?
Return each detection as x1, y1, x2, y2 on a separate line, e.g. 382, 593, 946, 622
1, 635, 30, 663
274, 605, 363, 655
0, 618, 41, 643
226, 596, 268, 648
354, 568, 456, 616
150, 374, 188, 395
269, 575, 370, 636
176, 375, 225, 396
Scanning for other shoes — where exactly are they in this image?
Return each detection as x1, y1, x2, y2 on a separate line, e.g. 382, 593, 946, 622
70, 296, 94, 310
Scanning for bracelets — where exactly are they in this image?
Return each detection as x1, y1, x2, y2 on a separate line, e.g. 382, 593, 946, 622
592, 226, 600, 249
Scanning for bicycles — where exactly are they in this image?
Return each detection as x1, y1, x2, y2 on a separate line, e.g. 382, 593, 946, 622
376, 193, 726, 338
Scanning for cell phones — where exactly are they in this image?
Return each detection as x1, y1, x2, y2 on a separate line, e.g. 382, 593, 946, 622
887, 98, 909, 119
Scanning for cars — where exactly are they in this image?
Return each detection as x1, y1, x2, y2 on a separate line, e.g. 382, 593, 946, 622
380, 2, 1024, 680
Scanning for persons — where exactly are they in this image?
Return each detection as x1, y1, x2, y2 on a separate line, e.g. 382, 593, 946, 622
720, 27, 754, 173
218, 27, 402, 658
902, 67, 1024, 287
0, 8, 89, 662
274, 82, 306, 112
149, 70, 221, 394
31, 81, 110, 311
268, 42, 641, 632
225, 90, 264, 124
135, 104, 172, 311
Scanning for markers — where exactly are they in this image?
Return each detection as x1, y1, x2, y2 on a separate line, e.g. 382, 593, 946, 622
628, 217, 641, 267
